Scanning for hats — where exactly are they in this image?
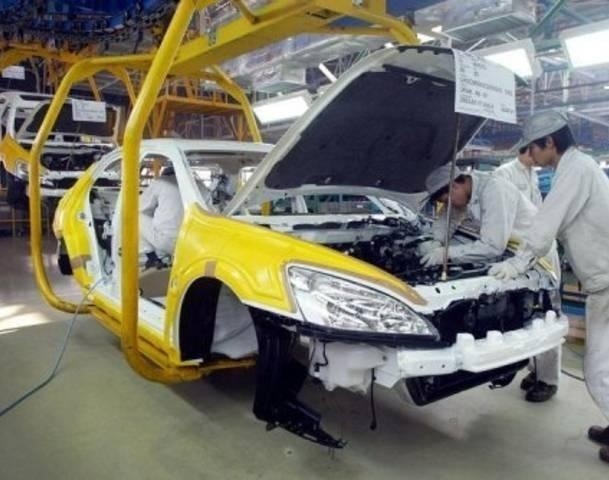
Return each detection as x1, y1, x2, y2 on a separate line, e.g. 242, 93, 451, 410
519, 111, 567, 149
420, 162, 461, 202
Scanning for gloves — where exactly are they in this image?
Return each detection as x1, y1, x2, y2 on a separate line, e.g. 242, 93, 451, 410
415, 240, 452, 272
488, 261, 518, 282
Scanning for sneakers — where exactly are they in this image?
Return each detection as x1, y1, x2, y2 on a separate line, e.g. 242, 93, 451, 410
588, 424, 609, 462
520, 369, 557, 402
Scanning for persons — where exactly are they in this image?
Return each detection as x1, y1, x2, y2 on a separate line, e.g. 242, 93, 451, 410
139, 167, 183, 269
